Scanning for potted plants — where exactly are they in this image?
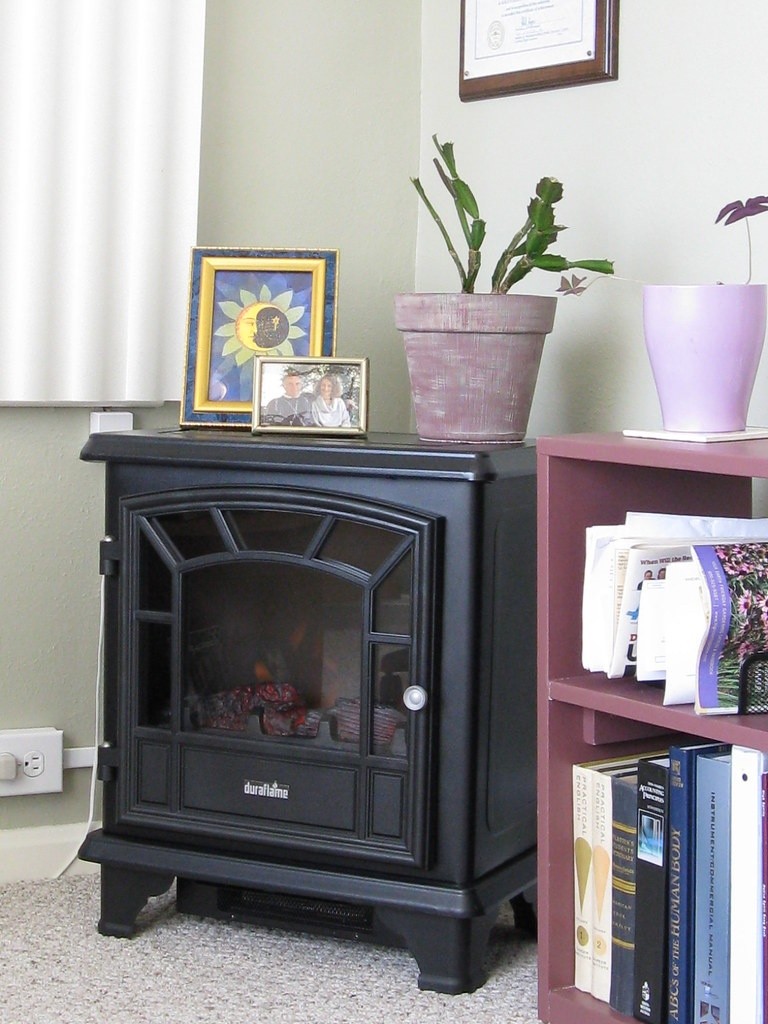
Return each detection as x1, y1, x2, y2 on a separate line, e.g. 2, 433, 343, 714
392, 135, 616, 445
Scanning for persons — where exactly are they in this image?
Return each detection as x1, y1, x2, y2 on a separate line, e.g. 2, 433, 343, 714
637, 569, 656, 590
311, 373, 352, 427
657, 568, 666, 579
265, 371, 317, 427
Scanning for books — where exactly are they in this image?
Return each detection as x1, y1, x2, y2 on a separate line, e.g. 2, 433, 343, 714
576, 507, 768, 716
571, 736, 768, 1024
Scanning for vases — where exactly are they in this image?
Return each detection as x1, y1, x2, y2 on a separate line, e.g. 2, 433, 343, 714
642, 284, 768, 432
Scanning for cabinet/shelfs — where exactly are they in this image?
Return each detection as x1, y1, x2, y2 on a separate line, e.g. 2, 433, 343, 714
84, 427, 538, 992
536, 430, 768, 1024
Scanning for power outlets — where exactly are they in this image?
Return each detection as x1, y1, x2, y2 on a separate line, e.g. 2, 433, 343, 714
0, 728, 64, 797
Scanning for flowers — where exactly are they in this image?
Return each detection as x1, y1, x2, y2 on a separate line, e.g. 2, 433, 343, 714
556, 194, 768, 298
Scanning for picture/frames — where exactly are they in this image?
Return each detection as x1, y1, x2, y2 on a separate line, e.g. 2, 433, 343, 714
252, 354, 370, 436
179, 246, 341, 430
458, 0, 619, 103
